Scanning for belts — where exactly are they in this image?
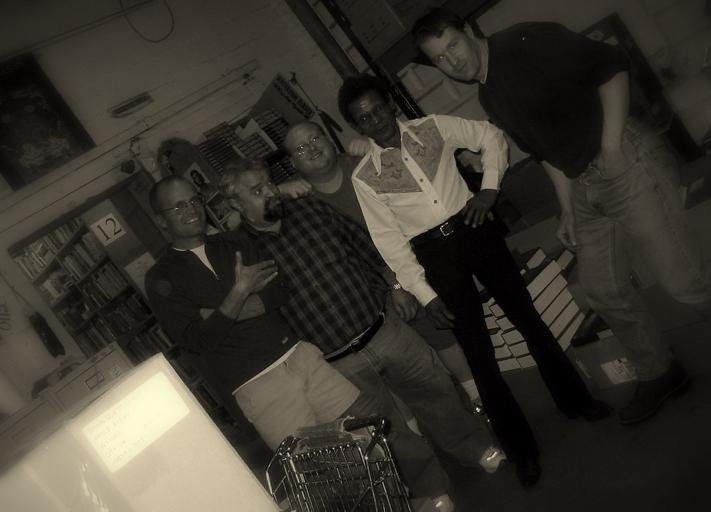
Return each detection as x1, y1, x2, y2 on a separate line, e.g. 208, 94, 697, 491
413, 213, 460, 247
325, 310, 386, 363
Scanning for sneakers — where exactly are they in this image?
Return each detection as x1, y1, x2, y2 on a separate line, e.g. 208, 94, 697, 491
429, 495, 454, 511
477, 446, 507, 474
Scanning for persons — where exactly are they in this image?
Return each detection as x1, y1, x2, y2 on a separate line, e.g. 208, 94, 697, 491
142, 5, 710, 512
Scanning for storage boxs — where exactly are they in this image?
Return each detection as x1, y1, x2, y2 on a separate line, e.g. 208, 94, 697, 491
504, 202, 561, 257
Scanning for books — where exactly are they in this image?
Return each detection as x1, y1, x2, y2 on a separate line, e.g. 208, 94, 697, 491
10, 216, 233, 426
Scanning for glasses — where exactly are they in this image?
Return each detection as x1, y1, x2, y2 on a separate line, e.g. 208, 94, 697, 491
294, 135, 320, 157
160, 196, 204, 216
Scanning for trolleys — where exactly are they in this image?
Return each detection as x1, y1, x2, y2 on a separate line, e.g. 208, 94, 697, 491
265, 414, 411, 512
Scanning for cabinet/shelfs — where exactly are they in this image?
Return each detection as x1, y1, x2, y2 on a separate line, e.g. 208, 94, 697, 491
11, 189, 245, 430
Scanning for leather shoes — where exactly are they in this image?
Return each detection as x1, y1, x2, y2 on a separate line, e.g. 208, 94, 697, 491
512, 450, 542, 487
618, 366, 689, 425
570, 399, 613, 422
